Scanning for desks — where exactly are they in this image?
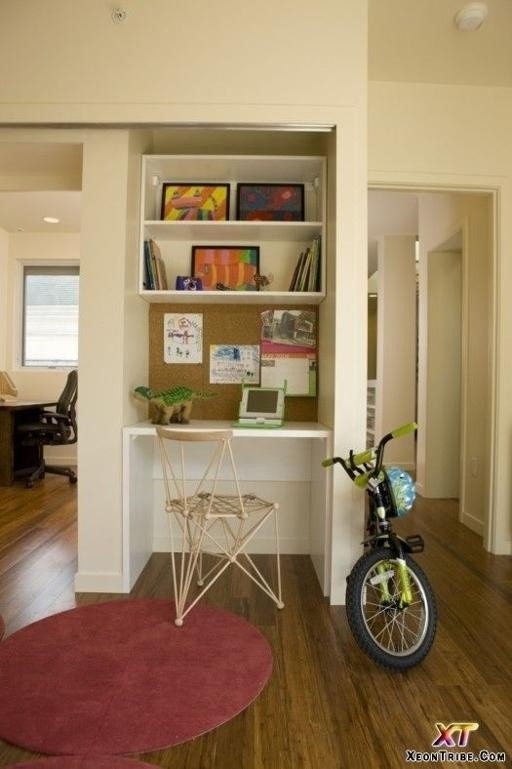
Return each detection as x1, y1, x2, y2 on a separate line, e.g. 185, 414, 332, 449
123, 416, 332, 601
0, 397, 65, 490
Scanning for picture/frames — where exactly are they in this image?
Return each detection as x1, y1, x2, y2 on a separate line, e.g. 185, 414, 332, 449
235, 181, 306, 221
189, 244, 261, 291
160, 180, 231, 222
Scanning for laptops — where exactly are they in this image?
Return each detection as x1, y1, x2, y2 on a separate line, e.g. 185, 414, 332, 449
238, 388, 285, 426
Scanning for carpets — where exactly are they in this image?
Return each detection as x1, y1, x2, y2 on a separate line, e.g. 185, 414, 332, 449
0, 594, 276, 757
0, 751, 165, 769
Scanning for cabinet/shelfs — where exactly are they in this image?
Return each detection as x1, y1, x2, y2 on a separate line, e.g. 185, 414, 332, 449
139, 149, 330, 306
368, 384, 416, 471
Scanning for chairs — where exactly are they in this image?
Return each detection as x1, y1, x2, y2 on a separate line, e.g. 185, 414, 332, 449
151, 422, 286, 627
21, 368, 79, 487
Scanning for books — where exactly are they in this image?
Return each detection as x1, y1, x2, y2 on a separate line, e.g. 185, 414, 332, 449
289, 235, 320, 292
145, 239, 167, 289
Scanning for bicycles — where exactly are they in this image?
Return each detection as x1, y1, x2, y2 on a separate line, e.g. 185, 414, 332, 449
319, 416, 438, 672
